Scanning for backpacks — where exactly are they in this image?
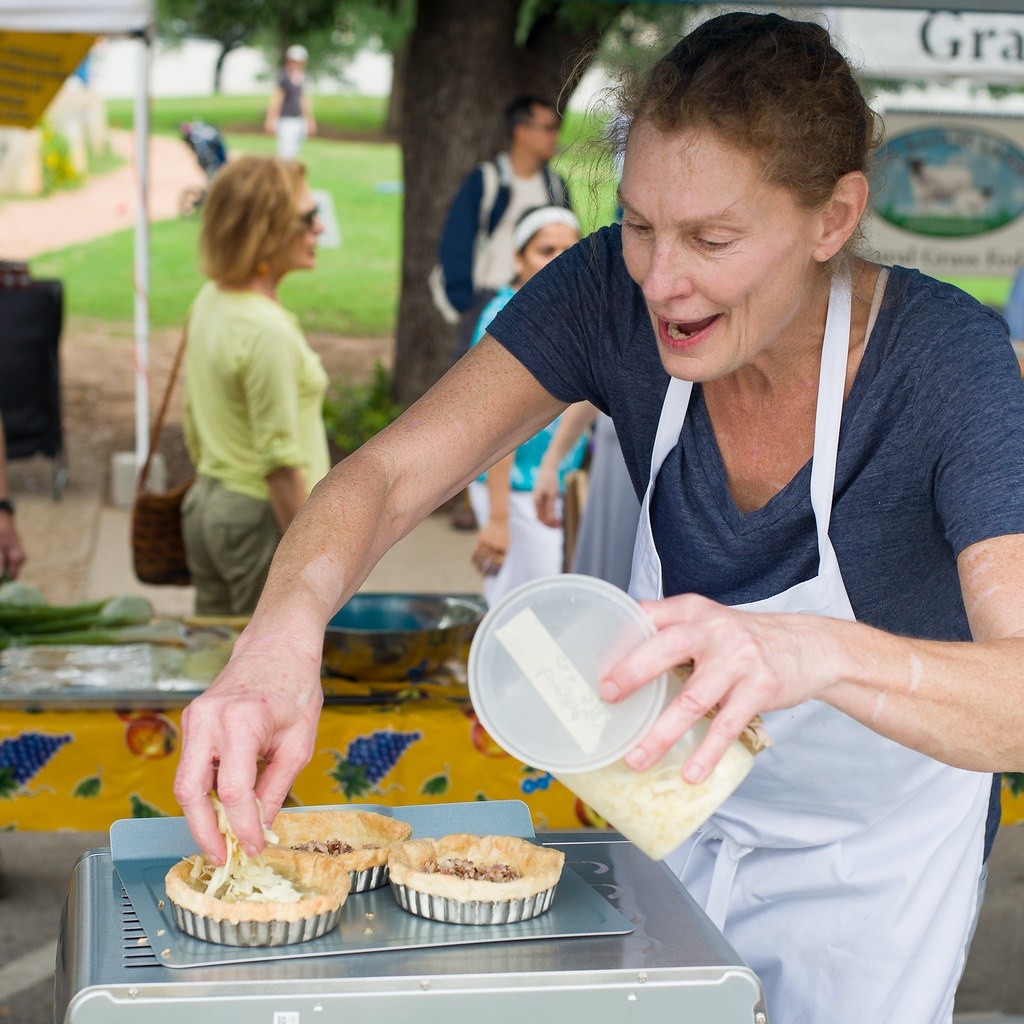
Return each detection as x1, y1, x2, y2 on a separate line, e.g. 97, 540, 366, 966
427, 161, 565, 326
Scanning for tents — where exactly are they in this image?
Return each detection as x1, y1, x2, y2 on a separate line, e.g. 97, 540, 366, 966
0, 0, 159, 456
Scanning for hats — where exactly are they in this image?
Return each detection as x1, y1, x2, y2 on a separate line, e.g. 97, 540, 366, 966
285, 44, 309, 62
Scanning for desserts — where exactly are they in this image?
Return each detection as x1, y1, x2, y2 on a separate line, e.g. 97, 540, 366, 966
164, 808, 565, 947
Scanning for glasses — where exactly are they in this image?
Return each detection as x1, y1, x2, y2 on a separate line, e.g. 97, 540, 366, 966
297, 204, 318, 225
518, 118, 558, 134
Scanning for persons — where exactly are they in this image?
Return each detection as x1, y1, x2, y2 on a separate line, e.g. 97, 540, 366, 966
171, 12, 1024, 1024
180, 155, 333, 620
428, 94, 575, 529
0, 409, 28, 581
465, 204, 590, 614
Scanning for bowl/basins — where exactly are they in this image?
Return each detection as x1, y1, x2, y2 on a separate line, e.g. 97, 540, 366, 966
324, 591, 488, 682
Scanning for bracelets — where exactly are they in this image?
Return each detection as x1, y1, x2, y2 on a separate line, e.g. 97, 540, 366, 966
0, 498, 17, 513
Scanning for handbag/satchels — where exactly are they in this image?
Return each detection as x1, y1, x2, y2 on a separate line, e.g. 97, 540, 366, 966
132, 478, 192, 582
